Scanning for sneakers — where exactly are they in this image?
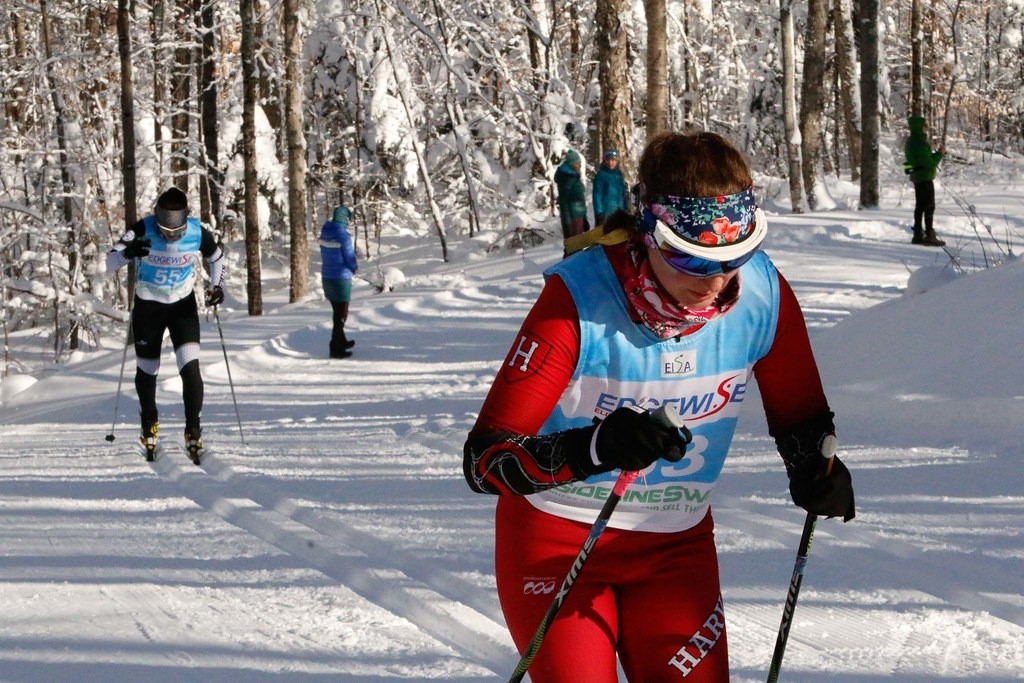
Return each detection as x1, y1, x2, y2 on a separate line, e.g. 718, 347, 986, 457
184, 426, 202, 451
141, 415, 159, 450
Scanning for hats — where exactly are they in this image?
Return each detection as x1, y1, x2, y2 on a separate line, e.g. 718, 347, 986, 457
335, 207, 351, 226
566, 150, 582, 165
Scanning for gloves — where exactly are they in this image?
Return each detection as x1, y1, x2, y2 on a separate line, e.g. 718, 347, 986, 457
207, 286, 224, 306
791, 458, 856, 523
124, 239, 151, 258
565, 407, 692, 473
583, 218, 590, 232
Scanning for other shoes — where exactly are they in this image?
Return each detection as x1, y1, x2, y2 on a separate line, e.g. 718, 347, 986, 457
329, 351, 352, 358
346, 340, 355, 348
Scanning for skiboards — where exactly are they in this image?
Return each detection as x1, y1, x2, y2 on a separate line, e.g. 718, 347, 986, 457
145, 449, 202, 466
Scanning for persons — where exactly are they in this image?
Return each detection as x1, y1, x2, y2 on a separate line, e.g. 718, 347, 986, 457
904, 117, 949, 247
105, 187, 225, 453
318, 207, 357, 358
592, 151, 632, 227
554, 150, 590, 259
462, 131, 856, 683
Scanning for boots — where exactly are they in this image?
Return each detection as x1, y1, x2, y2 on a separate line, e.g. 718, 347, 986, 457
923, 230, 946, 246
910, 227, 924, 245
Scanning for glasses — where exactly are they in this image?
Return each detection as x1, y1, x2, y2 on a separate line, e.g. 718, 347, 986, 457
653, 206, 768, 279
923, 123, 927, 128
605, 151, 617, 157
157, 223, 187, 236
